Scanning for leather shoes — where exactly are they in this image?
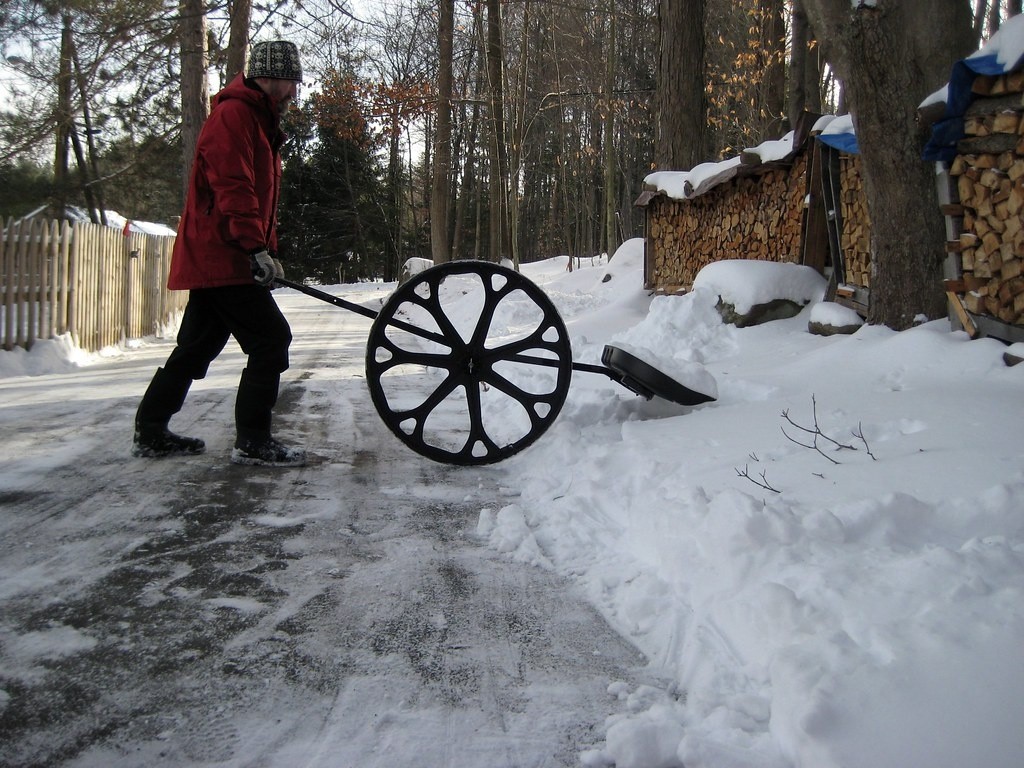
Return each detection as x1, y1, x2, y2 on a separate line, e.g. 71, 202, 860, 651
131, 431, 205, 458
232, 439, 306, 466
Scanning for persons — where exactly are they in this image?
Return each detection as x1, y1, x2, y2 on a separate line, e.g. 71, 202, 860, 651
130, 38, 307, 468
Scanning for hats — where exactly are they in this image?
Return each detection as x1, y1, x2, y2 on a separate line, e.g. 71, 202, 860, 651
247, 41, 302, 82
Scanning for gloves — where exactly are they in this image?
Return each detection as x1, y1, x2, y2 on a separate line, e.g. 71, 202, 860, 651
250, 246, 284, 288
